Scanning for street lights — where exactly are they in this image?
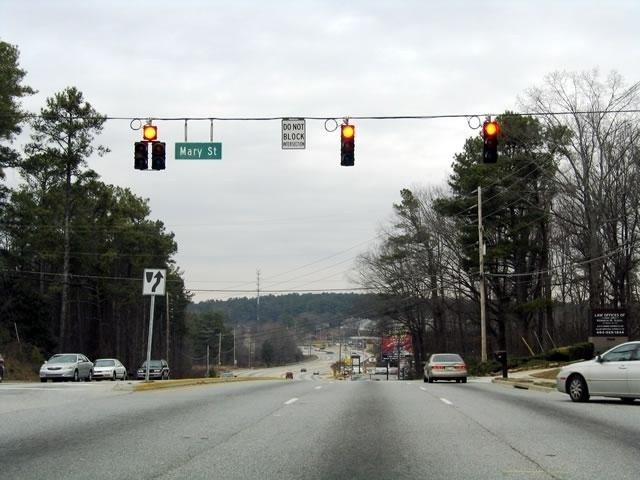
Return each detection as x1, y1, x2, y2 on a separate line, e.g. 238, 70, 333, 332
336, 342, 342, 374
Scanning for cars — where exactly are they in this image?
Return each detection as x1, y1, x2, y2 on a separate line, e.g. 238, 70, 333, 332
93, 357, 128, 381
555, 338, 640, 405
0, 354, 5, 383
38, 352, 95, 382
422, 351, 469, 384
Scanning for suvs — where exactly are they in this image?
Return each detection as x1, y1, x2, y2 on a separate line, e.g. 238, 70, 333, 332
136, 358, 171, 380
285, 372, 293, 379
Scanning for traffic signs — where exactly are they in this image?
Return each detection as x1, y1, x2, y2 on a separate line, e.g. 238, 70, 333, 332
175, 142, 222, 160
281, 119, 306, 150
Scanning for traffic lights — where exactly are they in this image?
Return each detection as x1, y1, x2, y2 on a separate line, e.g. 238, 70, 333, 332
340, 125, 355, 167
141, 125, 158, 141
151, 141, 166, 171
134, 141, 149, 170
482, 120, 499, 164
